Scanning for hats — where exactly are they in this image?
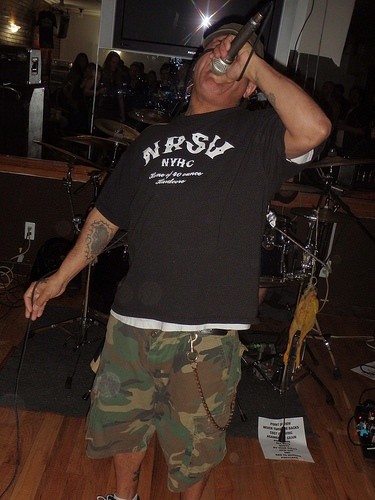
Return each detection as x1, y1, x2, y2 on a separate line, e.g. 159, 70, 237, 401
202, 23, 264, 59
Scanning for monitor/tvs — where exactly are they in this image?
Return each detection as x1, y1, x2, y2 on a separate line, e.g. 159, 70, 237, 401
98, 0, 297, 68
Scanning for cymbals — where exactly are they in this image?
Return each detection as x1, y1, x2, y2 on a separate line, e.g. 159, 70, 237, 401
93, 118, 140, 143
290, 204, 348, 224
59, 134, 114, 145
282, 182, 324, 193
130, 106, 168, 125
312, 149, 374, 169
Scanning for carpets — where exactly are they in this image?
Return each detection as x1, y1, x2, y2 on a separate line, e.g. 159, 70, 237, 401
0, 302, 314, 441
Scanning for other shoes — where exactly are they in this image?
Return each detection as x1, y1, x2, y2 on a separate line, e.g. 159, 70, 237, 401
96, 493, 140, 500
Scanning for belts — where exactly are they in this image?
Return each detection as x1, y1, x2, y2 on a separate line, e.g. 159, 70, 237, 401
191, 329, 231, 335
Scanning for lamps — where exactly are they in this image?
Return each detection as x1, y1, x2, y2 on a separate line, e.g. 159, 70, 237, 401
2, 15, 21, 33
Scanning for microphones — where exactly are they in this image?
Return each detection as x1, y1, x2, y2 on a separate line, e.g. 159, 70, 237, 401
212, 2, 273, 76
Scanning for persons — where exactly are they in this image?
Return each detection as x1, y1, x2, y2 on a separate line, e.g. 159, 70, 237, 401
67, 51, 160, 122
23, 23, 332, 500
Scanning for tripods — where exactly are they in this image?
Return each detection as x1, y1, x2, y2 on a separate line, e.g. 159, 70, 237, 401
241, 191, 343, 445
25, 263, 108, 390
